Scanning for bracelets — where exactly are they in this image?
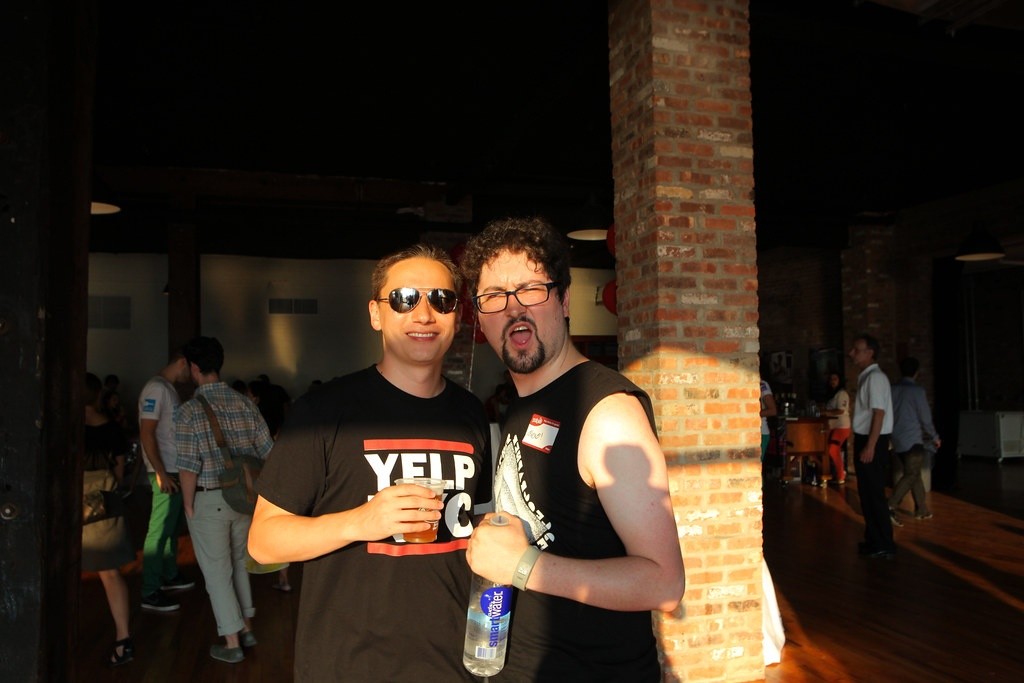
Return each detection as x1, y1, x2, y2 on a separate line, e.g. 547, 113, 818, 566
512, 545, 542, 591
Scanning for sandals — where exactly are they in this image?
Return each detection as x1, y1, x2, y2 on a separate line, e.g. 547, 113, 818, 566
104, 638, 135, 667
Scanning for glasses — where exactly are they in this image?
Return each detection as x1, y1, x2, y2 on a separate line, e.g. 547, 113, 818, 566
375, 287, 461, 314
473, 282, 560, 314
853, 348, 866, 353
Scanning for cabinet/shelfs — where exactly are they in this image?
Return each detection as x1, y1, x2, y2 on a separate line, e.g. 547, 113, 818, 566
777, 416, 838, 488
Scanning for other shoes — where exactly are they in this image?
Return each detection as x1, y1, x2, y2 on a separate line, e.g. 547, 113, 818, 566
837, 471, 847, 484
889, 511, 904, 527
915, 511, 934, 519
140, 589, 181, 611
160, 571, 195, 590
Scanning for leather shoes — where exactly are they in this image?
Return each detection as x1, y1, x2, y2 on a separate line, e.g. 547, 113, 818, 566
860, 546, 896, 561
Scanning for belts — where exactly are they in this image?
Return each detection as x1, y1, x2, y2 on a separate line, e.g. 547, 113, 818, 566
196, 486, 220, 493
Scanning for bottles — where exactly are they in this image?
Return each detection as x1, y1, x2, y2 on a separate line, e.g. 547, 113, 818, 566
806, 399, 821, 417
462, 515, 511, 678
774, 392, 797, 418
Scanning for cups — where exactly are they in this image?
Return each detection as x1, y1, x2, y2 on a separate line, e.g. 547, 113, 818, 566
394, 478, 446, 543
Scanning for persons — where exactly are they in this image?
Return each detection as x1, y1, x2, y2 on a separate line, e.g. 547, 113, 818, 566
178, 335, 292, 662
246, 245, 493, 683
458, 217, 686, 683
84, 370, 137, 665
760, 379, 777, 460
819, 372, 852, 483
889, 356, 941, 527
136, 351, 195, 610
848, 335, 897, 560
484, 370, 518, 419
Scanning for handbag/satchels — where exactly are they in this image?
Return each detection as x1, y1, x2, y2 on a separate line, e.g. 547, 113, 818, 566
82, 490, 112, 523
218, 454, 268, 514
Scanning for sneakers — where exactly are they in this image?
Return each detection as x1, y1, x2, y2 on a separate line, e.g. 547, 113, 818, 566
238, 631, 256, 647
210, 644, 244, 663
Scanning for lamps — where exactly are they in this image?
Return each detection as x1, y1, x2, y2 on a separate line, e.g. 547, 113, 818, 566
954, 215, 1007, 264
563, 189, 612, 241
88, 172, 122, 218
